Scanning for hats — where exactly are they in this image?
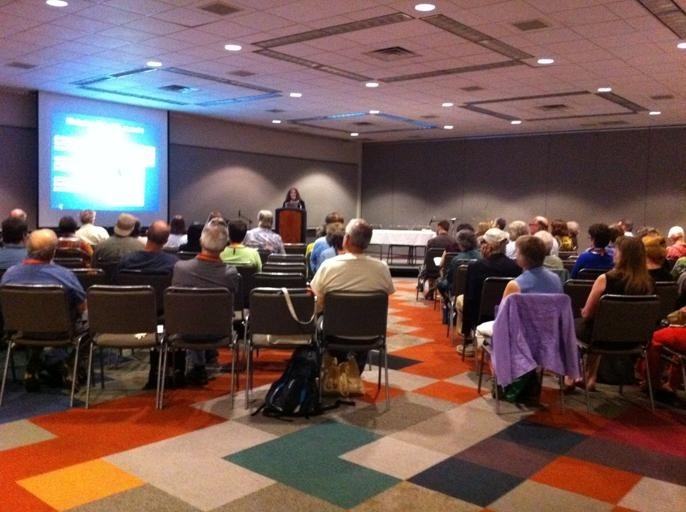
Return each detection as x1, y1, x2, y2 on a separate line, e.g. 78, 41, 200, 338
114, 213, 136, 236
479, 227, 510, 245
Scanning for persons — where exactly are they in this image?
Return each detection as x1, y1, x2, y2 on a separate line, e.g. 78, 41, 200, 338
305, 212, 396, 392
1, 209, 286, 383
280, 185, 305, 210
417, 216, 579, 400
570, 219, 686, 398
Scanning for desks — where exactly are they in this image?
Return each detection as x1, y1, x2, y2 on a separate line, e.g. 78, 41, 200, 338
369, 229, 438, 266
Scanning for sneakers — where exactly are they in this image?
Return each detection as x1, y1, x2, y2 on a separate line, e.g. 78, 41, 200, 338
456, 343, 477, 358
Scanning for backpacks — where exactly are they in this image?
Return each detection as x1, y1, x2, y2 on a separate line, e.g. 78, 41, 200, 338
249, 342, 366, 422
